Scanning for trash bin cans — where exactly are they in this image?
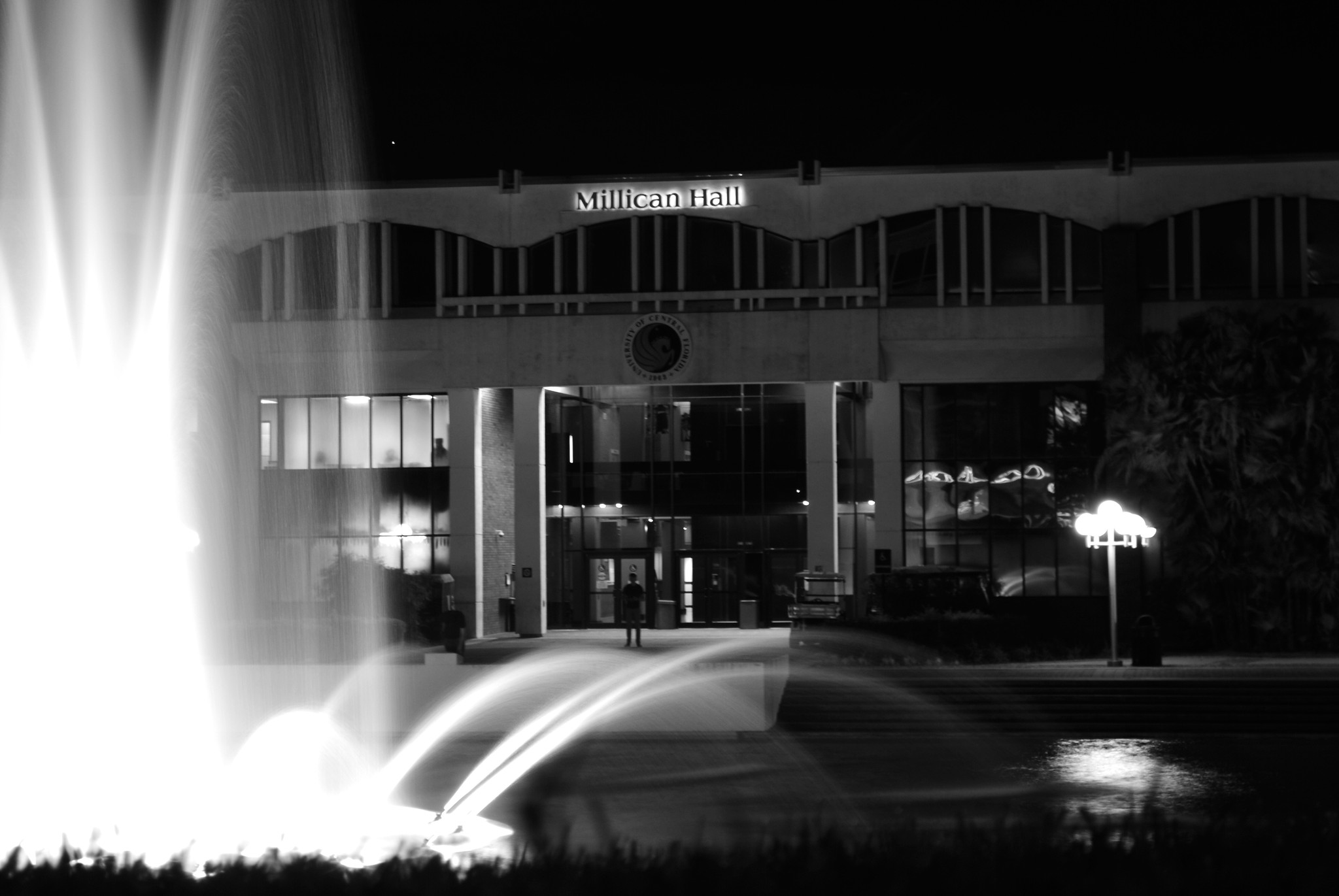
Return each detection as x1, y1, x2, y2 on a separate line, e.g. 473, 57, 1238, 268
654, 598, 676, 629
740, 599, 758, 627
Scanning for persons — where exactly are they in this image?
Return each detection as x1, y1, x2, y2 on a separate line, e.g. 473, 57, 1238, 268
621, 573, 644, 647
443, 602, 467, 656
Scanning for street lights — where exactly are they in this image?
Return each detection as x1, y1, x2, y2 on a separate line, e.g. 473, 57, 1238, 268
1074, 499, 1158, 667
